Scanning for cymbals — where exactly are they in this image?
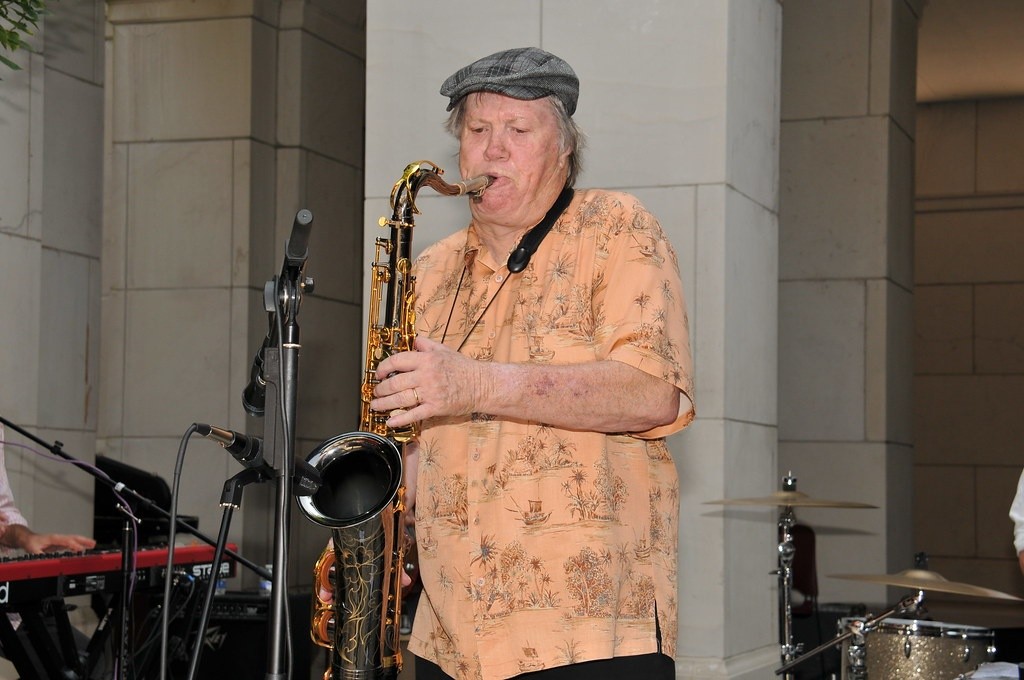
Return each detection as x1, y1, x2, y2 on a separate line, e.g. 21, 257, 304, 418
825, 568, 1023, 601
701, 492, 880, 509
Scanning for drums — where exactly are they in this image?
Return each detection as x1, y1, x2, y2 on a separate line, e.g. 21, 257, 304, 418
836, 617, 995, 680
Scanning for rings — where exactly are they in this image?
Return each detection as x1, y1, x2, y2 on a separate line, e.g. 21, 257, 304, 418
411, 386, 424, 406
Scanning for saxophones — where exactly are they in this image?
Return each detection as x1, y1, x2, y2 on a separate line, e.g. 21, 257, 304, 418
295, 161, 495, 680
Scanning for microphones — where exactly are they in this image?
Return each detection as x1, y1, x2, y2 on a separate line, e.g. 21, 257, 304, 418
192, 423, 265, 469
241, 330, 271, 416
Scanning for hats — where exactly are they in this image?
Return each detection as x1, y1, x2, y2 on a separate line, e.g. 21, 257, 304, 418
438, 46, 580, 116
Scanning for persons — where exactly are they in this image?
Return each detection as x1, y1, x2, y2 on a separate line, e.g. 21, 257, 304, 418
0, 404, 99, 678
314, 46, 697, 680
1007, 448, 1024, 591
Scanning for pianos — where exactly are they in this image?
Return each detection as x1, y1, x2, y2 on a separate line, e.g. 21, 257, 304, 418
0, 543, 238, 679
